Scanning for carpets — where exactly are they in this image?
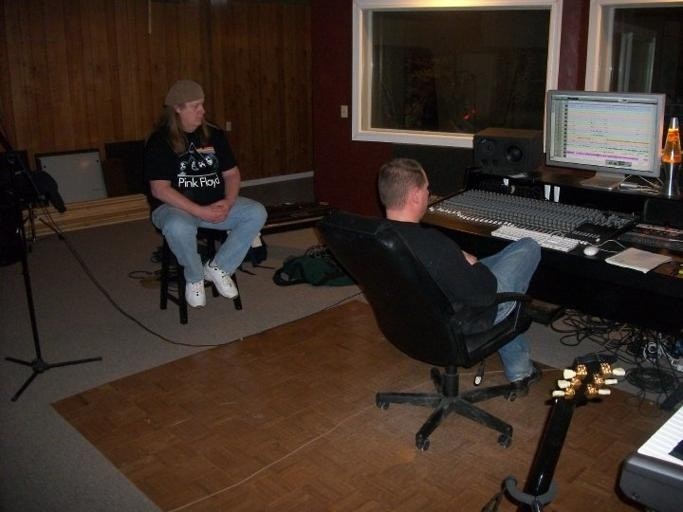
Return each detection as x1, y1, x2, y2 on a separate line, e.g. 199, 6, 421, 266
50, 300, 675, 512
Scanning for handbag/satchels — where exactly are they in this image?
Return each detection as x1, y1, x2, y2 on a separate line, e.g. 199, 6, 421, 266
273, 248, 356, 287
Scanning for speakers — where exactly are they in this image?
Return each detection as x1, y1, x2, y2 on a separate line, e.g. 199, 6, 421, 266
472, 127, 541, 173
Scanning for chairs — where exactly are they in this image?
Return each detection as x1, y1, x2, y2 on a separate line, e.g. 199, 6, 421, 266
314, 219, 540, 453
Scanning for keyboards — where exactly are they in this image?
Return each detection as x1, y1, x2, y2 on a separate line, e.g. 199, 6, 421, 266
491, 225, 580, 254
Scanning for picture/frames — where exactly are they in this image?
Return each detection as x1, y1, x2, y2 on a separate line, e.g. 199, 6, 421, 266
350, 0, 562, 150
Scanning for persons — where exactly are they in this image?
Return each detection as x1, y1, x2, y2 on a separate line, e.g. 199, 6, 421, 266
378, 158, 542, 398
140, 78, 268, 308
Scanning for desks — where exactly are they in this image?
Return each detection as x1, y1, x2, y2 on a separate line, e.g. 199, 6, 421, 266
510, 172, 683, 214
422, 190, 683, 336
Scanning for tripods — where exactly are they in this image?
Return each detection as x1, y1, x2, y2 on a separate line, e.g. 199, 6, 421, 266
4, 229, 104, 400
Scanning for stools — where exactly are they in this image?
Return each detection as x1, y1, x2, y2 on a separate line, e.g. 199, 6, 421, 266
157, 229, 244, 325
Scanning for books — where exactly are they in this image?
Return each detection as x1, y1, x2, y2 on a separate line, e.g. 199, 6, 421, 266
606, 248, 673, 274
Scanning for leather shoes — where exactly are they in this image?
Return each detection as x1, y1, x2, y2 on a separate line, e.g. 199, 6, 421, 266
512, 359, 541, 396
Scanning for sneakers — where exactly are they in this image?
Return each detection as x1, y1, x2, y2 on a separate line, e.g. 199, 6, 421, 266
203, 260, 238, 299
185, 278, 206, 308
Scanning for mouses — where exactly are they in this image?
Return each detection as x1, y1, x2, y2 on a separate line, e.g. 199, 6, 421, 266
584, 245, 600, 256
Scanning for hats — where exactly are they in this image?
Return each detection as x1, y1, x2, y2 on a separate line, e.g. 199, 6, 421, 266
164, 80, 203, 104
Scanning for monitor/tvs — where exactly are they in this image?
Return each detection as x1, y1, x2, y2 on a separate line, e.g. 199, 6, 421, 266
545, 90, 667, 191
